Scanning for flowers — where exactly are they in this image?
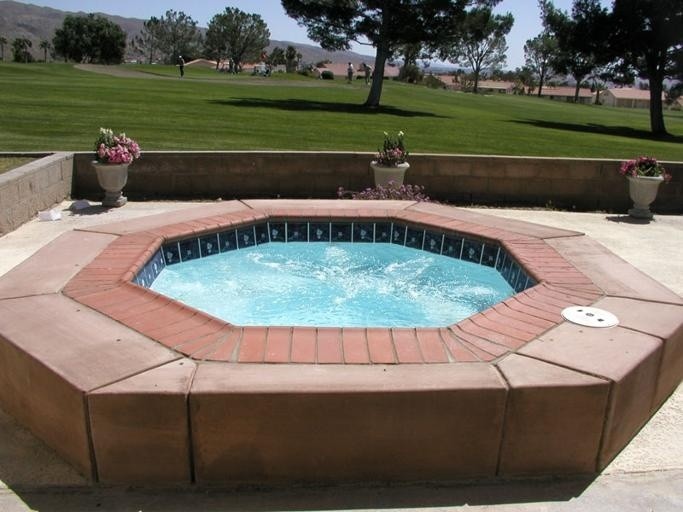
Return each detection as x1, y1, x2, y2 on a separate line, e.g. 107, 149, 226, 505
374, 130, 407, 166
617, 155, 672, 184
94, 126, 144, 165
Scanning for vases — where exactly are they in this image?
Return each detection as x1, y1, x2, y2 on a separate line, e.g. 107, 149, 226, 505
624, 172, 663, 221
370, 160, 411, 192
92, 161, 128, 208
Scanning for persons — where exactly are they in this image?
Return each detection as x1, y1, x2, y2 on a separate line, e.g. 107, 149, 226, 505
215, 56, 272, 77
177, 56, 184, 80
363, 64, 372, 86
346, 63, 353, 84
